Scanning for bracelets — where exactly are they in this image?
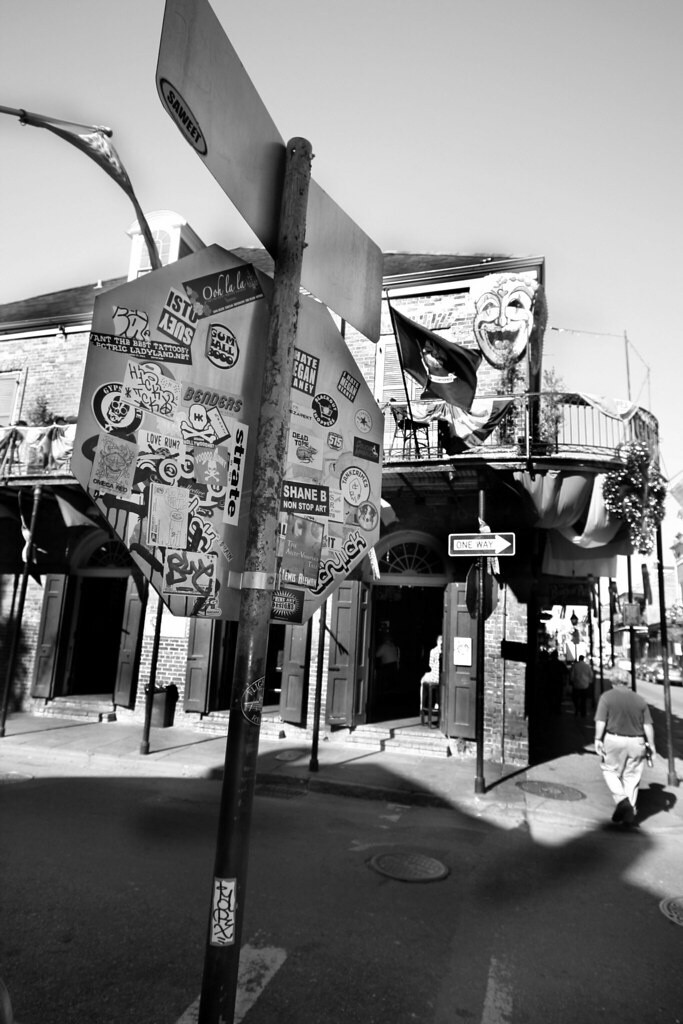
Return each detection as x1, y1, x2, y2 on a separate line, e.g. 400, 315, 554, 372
595, 738, 602, 740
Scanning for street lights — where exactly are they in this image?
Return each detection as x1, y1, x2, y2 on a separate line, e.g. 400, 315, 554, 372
569, 611, 579, 661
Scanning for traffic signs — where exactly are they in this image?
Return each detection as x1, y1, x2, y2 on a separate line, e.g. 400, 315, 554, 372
447, 532, 516, 557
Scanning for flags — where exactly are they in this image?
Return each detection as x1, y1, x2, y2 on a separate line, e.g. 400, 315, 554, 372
391, 305, 481, 412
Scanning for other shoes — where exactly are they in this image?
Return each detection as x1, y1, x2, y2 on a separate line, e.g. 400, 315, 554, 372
612, 799, 632, 821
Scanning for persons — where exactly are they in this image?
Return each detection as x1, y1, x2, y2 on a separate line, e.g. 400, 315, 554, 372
376, 635, 399, 670
537, 652, 560, 737
593, 669, 655, 821
551, 652, 570, 681
429, 635, 442, 671
570, 655, 593, 716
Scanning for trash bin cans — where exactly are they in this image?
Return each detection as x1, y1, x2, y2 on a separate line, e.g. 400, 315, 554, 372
143, 682, 179, 729
